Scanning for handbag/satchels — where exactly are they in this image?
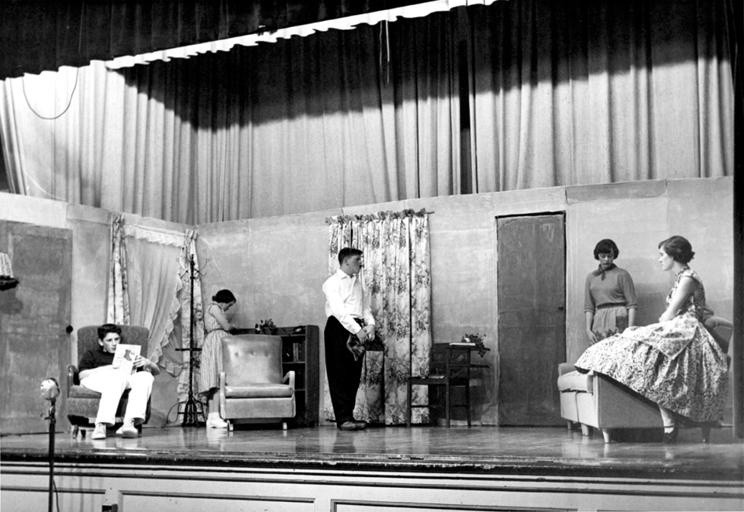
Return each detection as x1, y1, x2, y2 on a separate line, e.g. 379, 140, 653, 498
355, 333, 387, 352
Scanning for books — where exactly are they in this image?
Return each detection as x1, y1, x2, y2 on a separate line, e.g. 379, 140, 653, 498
112, 342, 141, 375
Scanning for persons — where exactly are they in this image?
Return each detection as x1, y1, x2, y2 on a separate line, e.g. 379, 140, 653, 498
196, 288, 236, 428
321, 245, 376, 431
574, 234, 730, 444
77, 321, 161, 440
582, 238, 640, 348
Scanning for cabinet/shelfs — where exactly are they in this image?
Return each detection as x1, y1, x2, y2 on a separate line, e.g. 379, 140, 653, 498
230, 325, 319, 431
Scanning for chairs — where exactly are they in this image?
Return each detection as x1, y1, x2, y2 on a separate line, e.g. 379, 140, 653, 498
65, 325, 152, 440
557, 316, 733, 445
407, 342, 473, 429
219, 333, 297, 431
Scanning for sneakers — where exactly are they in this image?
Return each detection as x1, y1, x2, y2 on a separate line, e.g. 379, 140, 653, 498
116, 424, 139, 438
91, 423, 106, 440
206, 418, 227, 429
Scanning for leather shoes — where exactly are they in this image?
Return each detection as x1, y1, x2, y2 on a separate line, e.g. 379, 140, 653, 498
662, 426, 679, 444
339, 421, 366, 433
